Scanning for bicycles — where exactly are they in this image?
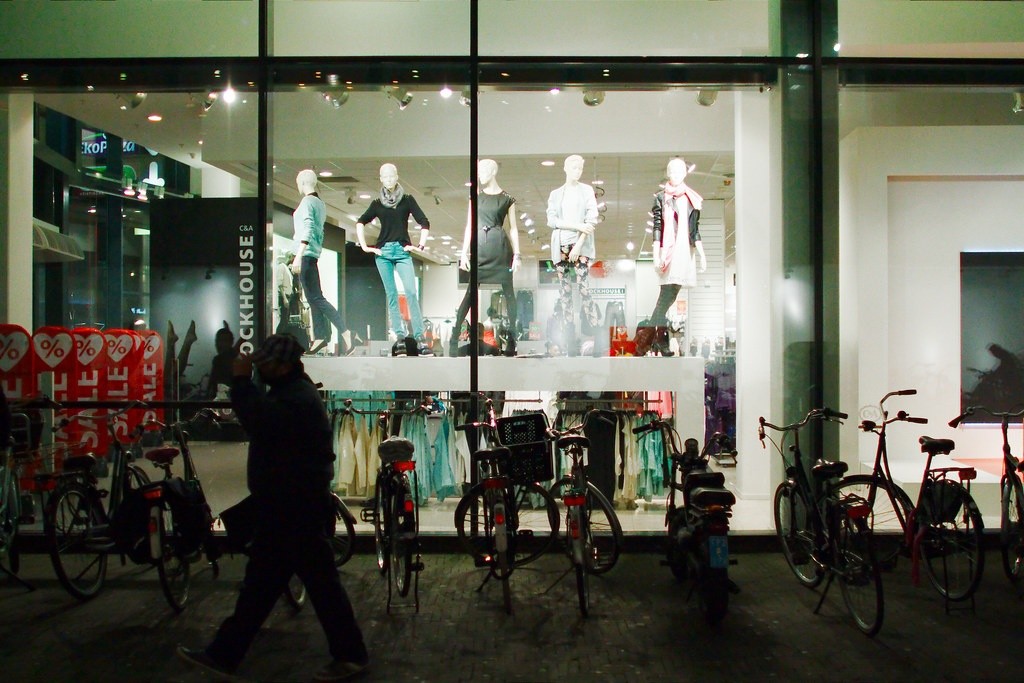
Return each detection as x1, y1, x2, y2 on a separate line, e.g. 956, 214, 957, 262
948, 405, 1024, 581
281, 408, 358, 615
342, 397, 434, 610
0, 393, 64, 590
815, 410, 986, 617
43, 397, 149, 602
758, 405, 884, 637
128, 407, 222, 605
543, 407, 638, 618
449, 390, 560, 617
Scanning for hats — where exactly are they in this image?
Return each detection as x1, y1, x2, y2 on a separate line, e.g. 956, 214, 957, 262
249, 335, 304, 368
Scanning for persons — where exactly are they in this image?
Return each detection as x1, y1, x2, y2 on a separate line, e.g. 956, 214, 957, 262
451, 322, 505, 421
547, 155, 600, 357
635, 159, 707, 357
450, 159, 523, 356
177, 333, 372, 683
293, 168, 363, 357
355, 163, 430, 355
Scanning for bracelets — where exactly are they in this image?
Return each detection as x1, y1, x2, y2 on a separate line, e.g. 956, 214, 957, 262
512, 253, 522, 260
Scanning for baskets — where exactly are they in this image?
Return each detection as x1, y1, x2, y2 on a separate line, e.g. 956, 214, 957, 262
495, 412, 554, 484
8, 410, 45, 458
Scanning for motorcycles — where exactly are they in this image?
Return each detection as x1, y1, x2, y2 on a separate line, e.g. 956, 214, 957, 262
632, 418, 739, 634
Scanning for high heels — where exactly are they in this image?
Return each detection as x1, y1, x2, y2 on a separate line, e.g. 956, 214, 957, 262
304, 339, 328, 356
345, 329, 363, 356
654, 340, 674, 357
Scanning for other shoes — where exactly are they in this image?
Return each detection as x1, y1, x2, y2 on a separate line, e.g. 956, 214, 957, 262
504, 337, 515, 356
416, 337, 435, 357
314, 657, 373, 681
450, 339, 459, 357
177, 645, 237, 681
395, 340, 407, 357
